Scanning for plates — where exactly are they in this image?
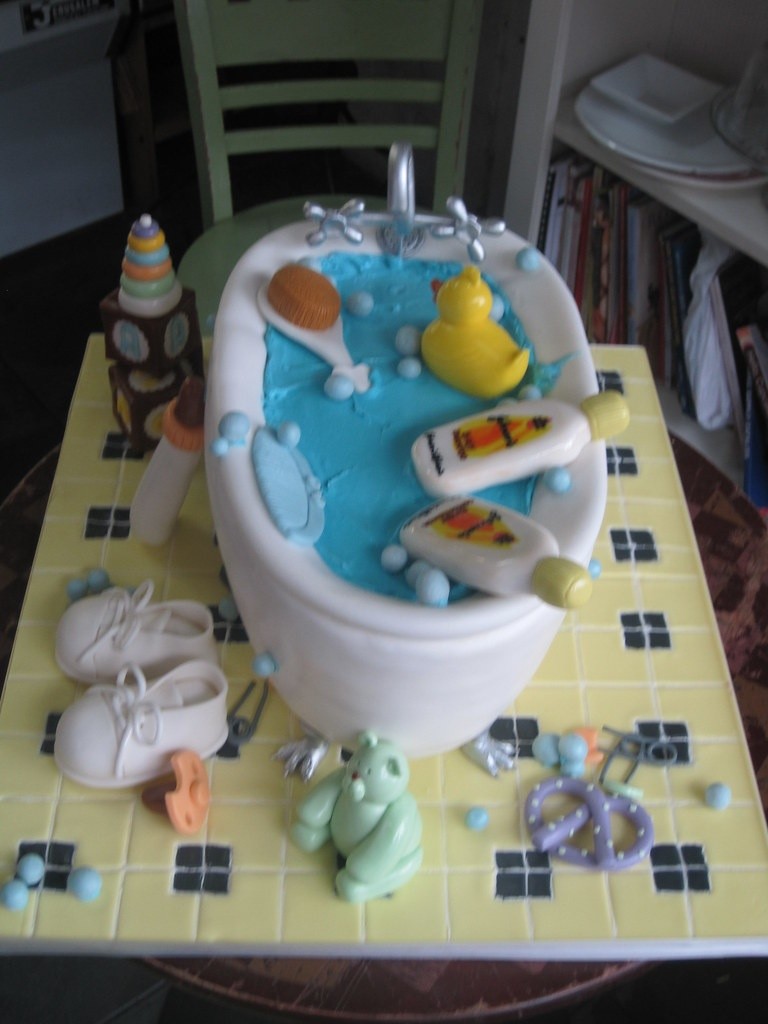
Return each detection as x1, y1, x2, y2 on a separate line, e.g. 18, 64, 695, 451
617, 154, 767, 190
575, 86, 750, 174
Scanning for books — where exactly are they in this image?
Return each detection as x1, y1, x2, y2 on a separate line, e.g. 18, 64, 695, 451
537, 155, 768, 510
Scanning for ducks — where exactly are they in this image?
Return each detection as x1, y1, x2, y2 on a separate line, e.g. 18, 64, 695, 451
423, 264, 530, 399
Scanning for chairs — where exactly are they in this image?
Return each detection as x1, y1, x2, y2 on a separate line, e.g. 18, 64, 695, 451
176, 0, 484, 334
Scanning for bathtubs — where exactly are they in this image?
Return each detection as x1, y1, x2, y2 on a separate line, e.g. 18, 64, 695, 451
204, 210, 610, 764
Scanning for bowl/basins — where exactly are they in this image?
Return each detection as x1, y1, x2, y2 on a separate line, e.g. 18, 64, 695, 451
592, 53, 722, 122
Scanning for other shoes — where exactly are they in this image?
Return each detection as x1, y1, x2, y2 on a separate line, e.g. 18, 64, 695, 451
54, 579, 214, 684
53, 657, 228, 790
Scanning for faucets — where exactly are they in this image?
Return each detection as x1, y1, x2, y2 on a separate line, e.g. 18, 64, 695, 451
382, 139, 420, 253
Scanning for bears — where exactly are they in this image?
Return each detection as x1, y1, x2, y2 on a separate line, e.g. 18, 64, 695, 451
288, 728, 422, 902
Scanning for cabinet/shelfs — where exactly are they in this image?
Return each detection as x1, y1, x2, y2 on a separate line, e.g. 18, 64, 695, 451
501, 2, 766, 538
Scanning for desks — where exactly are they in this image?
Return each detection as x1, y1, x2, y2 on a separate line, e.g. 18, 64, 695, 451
0, 330, 767, 964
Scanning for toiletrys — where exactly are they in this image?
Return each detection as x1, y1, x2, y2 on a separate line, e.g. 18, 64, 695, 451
410, 387, 631, 501
398, 491, 592, 611
